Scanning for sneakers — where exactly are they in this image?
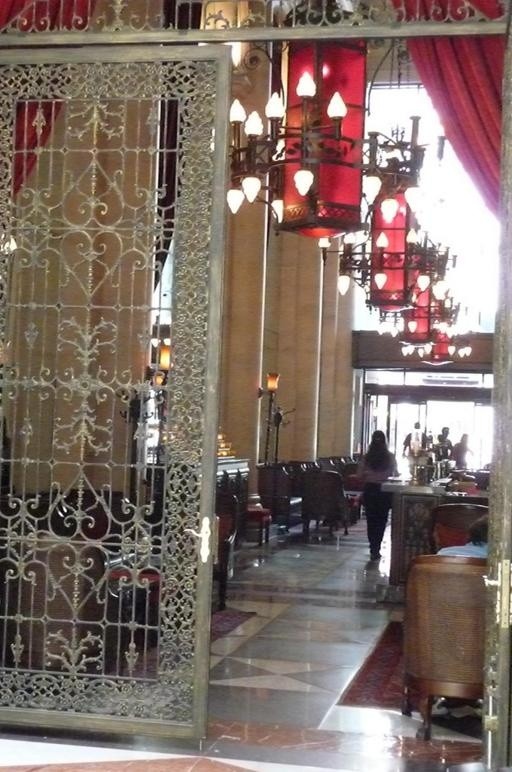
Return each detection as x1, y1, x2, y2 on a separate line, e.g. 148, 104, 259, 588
370, 552, 382, 561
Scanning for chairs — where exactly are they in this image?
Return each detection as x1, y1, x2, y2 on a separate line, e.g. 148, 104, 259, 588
257, 453, 364, 547
396, 552, 490, 747
0, 467, 274, 673
423, 501, 490, 558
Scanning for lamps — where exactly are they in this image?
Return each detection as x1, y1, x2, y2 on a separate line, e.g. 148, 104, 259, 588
262, 370, 282, 466
225, 31, 468, 365
151, 344, 173, 462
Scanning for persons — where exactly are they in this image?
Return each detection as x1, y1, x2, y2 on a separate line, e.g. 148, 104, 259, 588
401, 421, 475, 478
436, 514, 488, 559
355, 430, 399, 560
